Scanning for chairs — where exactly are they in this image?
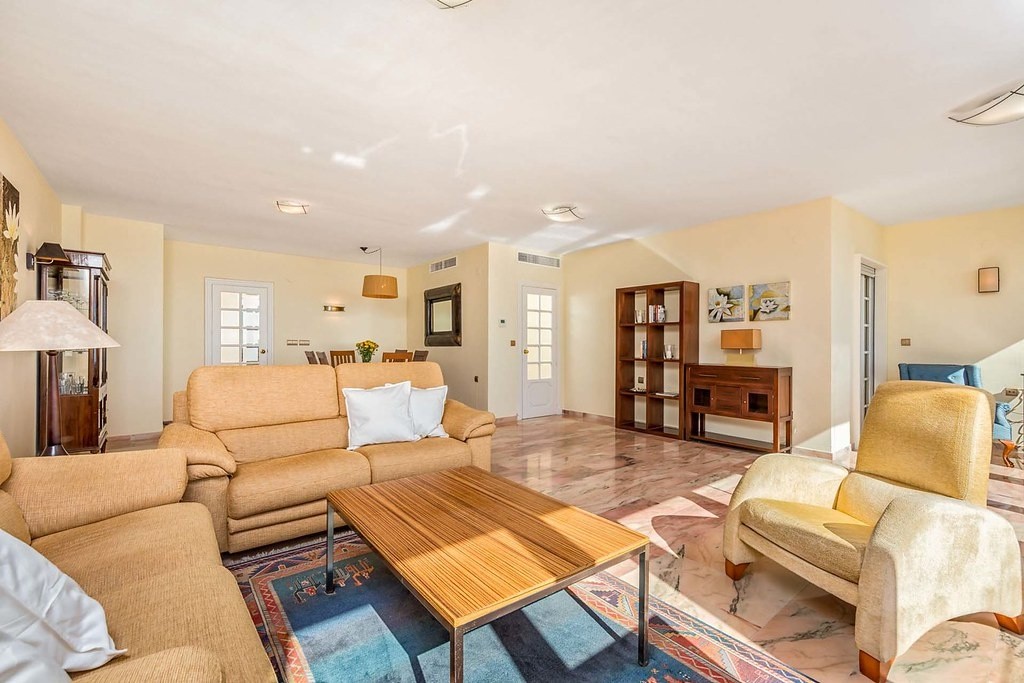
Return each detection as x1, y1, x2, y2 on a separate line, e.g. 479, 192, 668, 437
393, 349, 407, 362
413, 350, 429, 362
330, 350, 356, 368
305, 351, 318, 364
898, 363, 1015, 468
316, 352, 329, 365
382, 352, 413, 362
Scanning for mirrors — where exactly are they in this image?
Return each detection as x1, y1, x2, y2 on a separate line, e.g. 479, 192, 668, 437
424, 282, 462, 347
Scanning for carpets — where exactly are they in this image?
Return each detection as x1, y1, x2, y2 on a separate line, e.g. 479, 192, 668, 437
222, 529, 819, 683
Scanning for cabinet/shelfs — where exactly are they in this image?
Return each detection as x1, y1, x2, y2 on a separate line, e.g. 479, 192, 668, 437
38, 249, 113, 455
684, 362, 793, 456
615, 280, 700, 441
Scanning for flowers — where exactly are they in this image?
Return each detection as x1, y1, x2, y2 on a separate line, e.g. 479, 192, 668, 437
355, 340, 380, 363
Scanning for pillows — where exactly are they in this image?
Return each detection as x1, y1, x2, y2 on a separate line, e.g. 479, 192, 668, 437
0, 528, 129, 673
385, 382, 450, 438
341, 380, 421, 451
0, 631, 73, 683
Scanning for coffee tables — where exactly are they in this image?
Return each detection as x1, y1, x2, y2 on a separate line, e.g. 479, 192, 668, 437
325, 465, 651, 683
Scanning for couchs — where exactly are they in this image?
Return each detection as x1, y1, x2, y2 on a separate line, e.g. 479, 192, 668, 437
723, 379, 1024, 683
0, 430, 279, 683
158, 362, 497, 555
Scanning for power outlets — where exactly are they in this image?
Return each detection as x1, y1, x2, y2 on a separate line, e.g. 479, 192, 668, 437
901, 338, 911, 346
475, 376, 478, 382
1005, 388, 1018, 396
638, 376, 644, 384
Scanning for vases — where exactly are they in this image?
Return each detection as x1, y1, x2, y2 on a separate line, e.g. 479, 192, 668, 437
663, 344, 675, 359
634, 310, 645, 322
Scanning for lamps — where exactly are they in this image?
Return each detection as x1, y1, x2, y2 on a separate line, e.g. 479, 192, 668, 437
977, 267, 1000, 293
949, 83, 1024, 128
275, 200, 312, 215
323, 304, 345, 313
721, 329, 762, 365
26, 242, 70, 271
359, 247, 398, 299
0, 300, 121, 457
539, 203, 586, 223
